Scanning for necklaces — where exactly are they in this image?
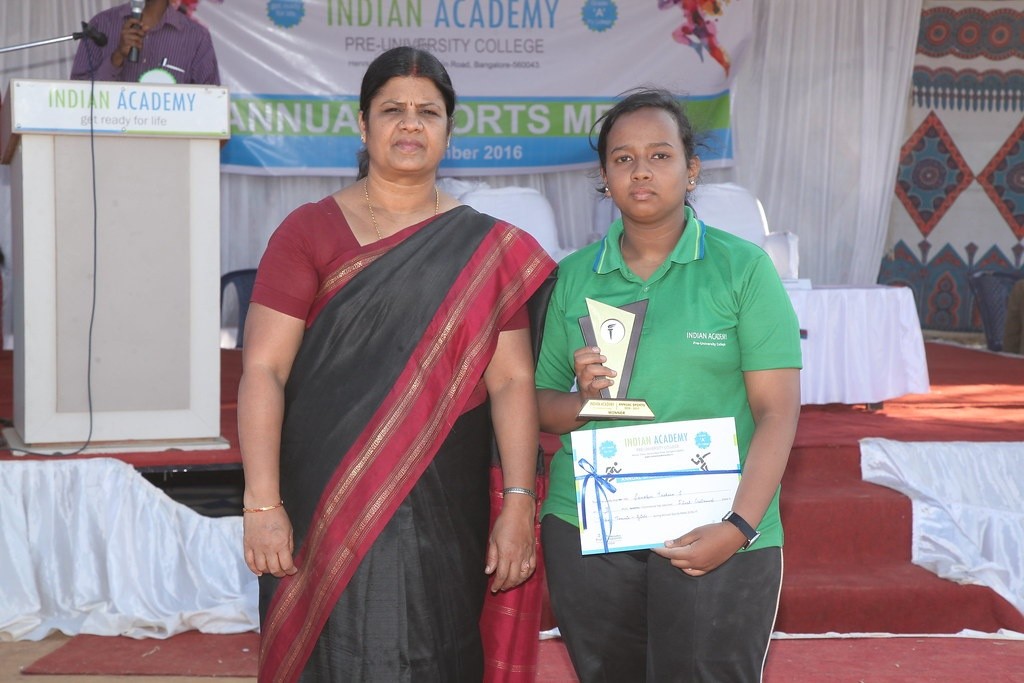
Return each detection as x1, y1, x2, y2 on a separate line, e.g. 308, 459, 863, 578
364, 177, 440, 239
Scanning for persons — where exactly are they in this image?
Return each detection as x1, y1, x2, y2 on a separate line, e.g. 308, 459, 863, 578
234, 46, 558, 683
535, 85, 804, 683
71, 1, 220, 86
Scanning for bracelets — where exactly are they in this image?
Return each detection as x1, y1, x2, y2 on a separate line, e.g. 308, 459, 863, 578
242, 502, 283, 514
503, 487, 536, 499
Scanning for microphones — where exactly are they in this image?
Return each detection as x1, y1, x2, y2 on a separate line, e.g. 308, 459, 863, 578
80, 20, 109, 47
126, 0, 147, 64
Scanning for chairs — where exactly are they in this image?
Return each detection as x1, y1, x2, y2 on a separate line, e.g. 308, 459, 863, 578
686, 185, 799, 287
456, 186, 560, 259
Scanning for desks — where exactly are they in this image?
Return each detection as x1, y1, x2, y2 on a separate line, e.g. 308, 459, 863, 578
781, 282, 930, 414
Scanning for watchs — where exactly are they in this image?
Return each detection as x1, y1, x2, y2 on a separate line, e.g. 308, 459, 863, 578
723, 510, 763, 551
